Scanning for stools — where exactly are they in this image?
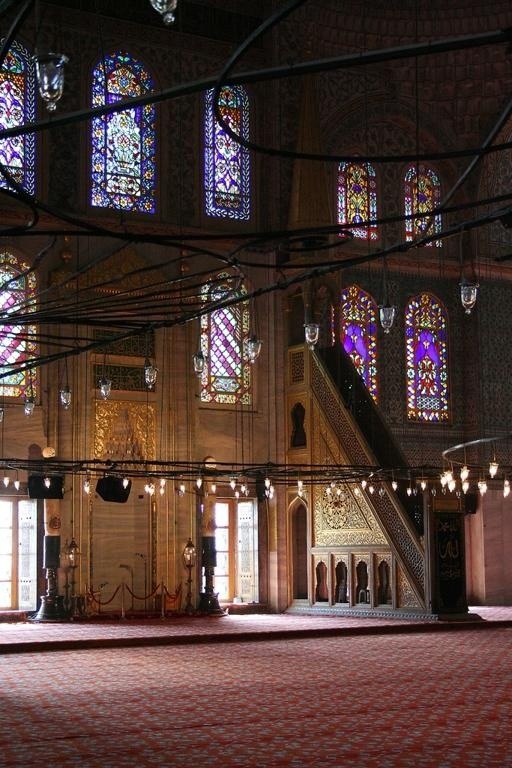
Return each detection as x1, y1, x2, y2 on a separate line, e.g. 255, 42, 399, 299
359, 589, 370, 603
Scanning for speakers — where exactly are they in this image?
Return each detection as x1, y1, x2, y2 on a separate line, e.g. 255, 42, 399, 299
256, 480, 266, 502
26, 473, 65, 499
95, 476, 132, 503
465, 493, 478, 514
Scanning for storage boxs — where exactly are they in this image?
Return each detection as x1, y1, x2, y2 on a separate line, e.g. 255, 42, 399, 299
154, 593, 179, 615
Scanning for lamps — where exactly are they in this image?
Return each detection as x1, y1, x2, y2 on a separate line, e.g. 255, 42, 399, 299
183, 537, 198, 616
149, 0, 178, 26
196, 536, 227, 617
25, 534, 68, 623
28, 0, 71, 112
68, 537, 80, 621
